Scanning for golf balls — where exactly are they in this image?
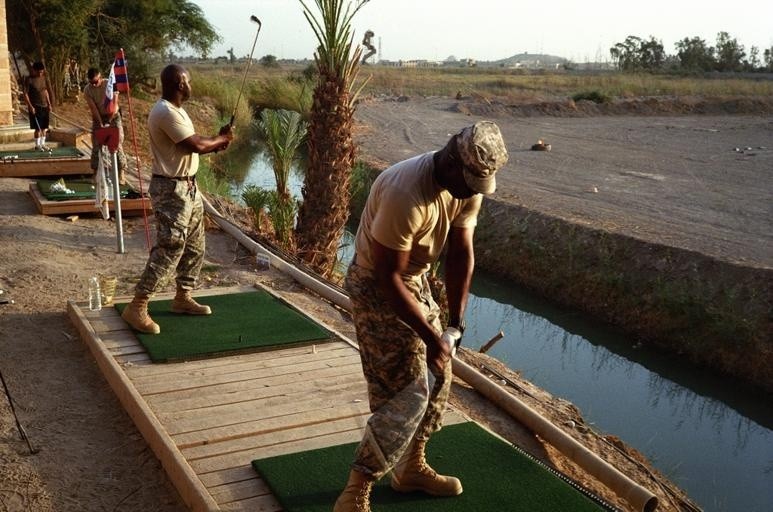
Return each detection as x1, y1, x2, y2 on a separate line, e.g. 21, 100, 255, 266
3, 155, 18, 159
49, 152, 51, 153
65, 188, 75, 194
90, 186, 95, 190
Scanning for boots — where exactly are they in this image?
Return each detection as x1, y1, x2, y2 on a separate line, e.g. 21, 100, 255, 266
334, 470, 376, 512
118, 169, 125, 185
171, 287, 211, 315
392, 438, 463, 496
121, 294, 160, 334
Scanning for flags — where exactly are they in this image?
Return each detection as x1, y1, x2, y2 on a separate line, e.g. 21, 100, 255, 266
102, 52, 129, 114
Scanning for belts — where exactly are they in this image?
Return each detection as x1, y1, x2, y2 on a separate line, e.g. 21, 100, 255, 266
153, 171, 197, 180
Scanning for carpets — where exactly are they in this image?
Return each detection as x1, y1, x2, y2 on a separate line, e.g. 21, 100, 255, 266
0, 143, 80, 158
250, 421, 607, 512
115, 289, 332, 364
37, 178, 139, 201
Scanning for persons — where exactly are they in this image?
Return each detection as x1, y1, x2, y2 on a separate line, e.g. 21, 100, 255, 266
331, 120, 507, 511
22, 62, 52, 153
82, 68, 124, 185
119, 60, 236, 334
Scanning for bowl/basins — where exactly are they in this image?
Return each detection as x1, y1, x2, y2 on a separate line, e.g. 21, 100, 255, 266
531, 144, 551, 151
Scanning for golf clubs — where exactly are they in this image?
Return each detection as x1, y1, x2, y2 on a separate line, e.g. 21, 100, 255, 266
229, 15, 262, 129
105, 146, 113, 186
34, 113, 53, 151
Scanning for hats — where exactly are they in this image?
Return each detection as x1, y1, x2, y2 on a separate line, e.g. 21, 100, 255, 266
457, 120, 508, 195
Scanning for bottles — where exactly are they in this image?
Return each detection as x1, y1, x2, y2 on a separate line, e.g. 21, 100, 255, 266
87, 278, 101, 311
107, 179, 113, 200
0, 155, 19, 161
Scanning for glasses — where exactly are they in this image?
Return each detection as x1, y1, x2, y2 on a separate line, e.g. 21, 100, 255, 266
88, 81, 99, 83
